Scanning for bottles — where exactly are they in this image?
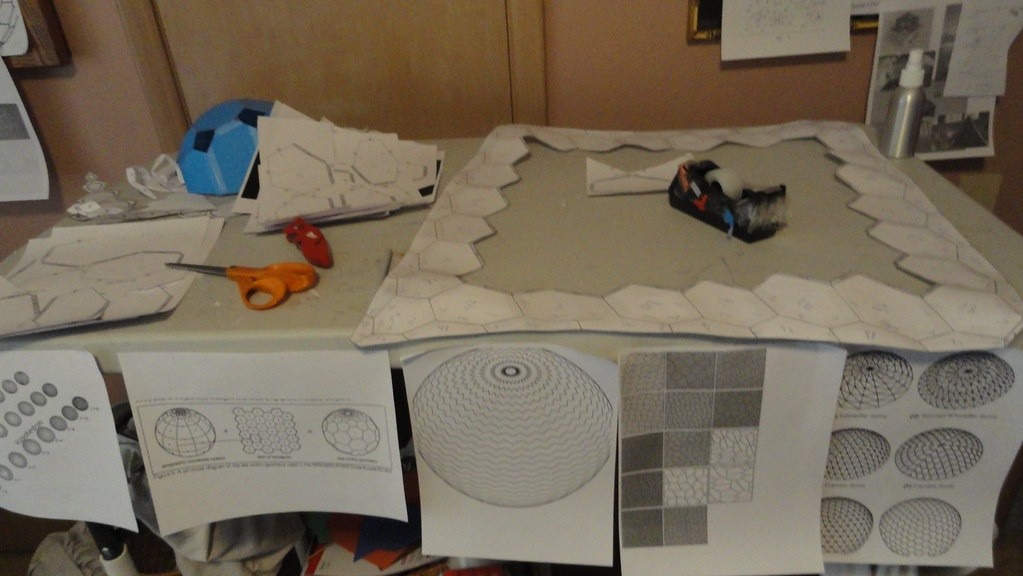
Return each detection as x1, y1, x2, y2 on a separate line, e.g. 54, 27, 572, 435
878, 46, 926, 159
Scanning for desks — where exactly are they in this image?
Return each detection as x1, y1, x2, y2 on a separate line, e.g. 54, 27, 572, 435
0, 123, 1023, 576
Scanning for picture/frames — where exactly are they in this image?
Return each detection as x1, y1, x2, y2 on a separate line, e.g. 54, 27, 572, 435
687, 0, 879, 45
0, 0, 72, 70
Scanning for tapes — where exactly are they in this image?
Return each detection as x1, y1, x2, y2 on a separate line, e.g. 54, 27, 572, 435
705, 168, 745, 201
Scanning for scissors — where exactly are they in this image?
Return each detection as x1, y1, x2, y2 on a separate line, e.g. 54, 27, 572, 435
165, 262, 319, 311
